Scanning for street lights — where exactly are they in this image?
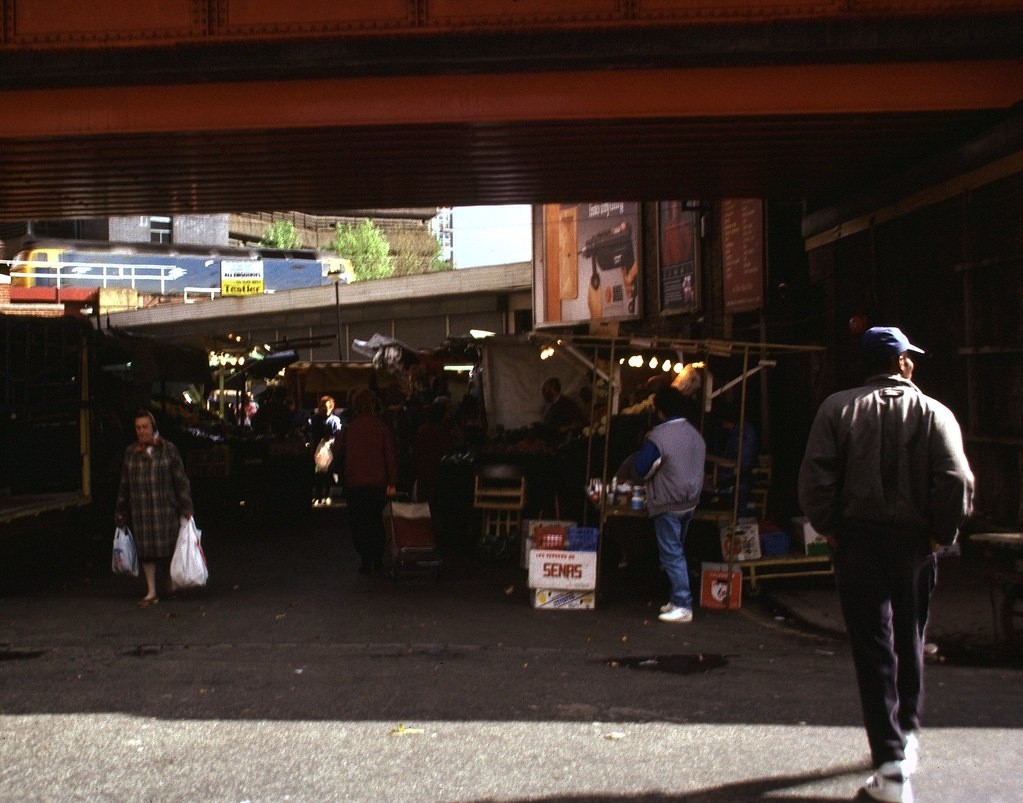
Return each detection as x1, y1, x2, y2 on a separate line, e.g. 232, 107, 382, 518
328, 267, 344, 364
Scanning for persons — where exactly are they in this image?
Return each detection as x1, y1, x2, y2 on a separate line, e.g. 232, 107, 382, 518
798, 327, 976, 803
635, 386, 706, 623
256, 387, 474, 575
714, 404, 757, 508
541, 378, 593, 468
613, 450, 644, 568
116, 409, 194, 606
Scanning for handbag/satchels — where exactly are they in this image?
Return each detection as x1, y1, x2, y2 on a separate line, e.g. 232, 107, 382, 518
169, 514, 209, 588
111, 524, 139, 578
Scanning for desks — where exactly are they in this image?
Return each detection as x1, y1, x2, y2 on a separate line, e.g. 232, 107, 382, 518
738, 555, 834, 590
970, 531, 1023, 646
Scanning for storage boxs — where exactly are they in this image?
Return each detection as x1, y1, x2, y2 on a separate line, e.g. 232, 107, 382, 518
699, 561, 742, 611
720, 516, 829, 563
521, 518, 600, 609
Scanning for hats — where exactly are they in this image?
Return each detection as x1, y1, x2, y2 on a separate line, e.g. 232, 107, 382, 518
862, 327, 925, 357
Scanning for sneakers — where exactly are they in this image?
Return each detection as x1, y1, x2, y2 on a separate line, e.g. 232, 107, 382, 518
660, 602, 678, 613
658, 607, 693, 622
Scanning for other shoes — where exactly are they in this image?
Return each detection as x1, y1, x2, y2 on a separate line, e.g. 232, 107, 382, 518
136, 596, 158, 606
374, 559, 384, 569
168, 591, 177, 600
358, 548, 373, 573
865, 773, 913, 803
901, 734, 918, 774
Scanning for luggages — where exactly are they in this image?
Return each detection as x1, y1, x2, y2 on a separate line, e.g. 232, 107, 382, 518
381, 491, 443, 580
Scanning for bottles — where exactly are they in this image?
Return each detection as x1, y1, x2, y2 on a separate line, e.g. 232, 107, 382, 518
586, 476, 645, 512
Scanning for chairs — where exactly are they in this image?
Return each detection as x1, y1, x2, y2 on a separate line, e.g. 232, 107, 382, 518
750, 453, 774, 518
